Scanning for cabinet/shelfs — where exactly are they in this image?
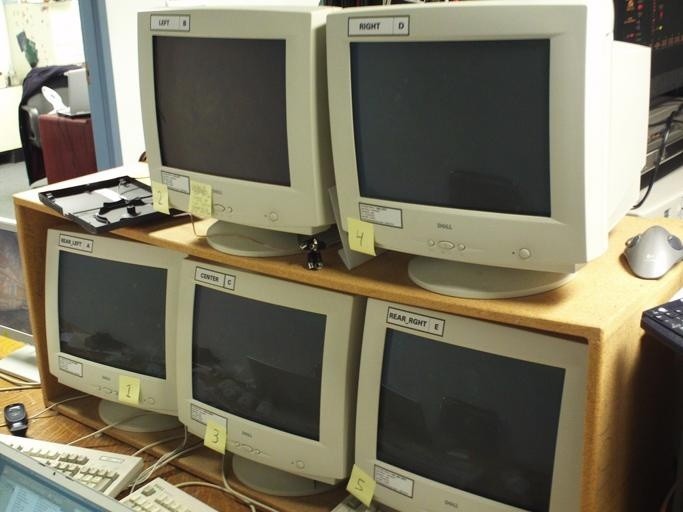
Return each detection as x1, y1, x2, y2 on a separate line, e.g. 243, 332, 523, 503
11, 163, 682, 510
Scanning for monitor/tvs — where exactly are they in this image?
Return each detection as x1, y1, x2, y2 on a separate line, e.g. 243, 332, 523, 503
44, 226, 193, 432
136, 7, 340, 257
351, 298, 592, 511
175, 259, 363, 499
324, 4, 654, 298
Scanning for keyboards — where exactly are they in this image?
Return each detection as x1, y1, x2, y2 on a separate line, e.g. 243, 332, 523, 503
118, 477, 220, 512
641, 299, 683, 353
0, 432, 146, 501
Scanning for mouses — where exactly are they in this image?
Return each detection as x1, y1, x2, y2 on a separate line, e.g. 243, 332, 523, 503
624, 223, 683, 280
